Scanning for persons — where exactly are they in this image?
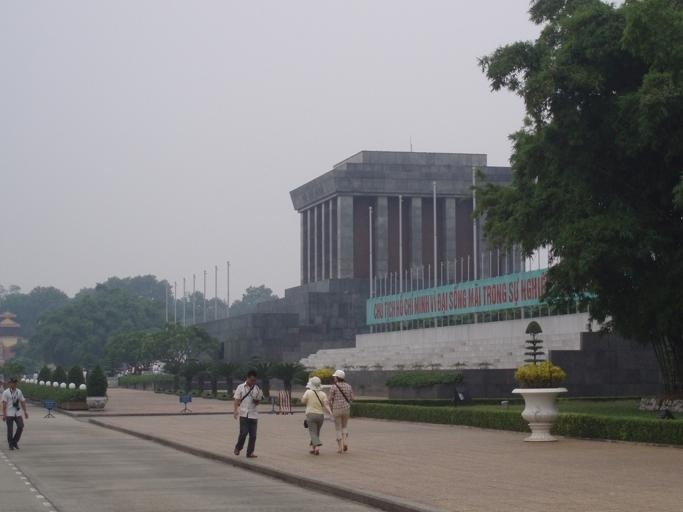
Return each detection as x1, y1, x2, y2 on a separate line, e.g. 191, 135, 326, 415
299, 376, 333, 454
0, 378, 28, 450
328, 369, 353, 453
230, 369, 263, 457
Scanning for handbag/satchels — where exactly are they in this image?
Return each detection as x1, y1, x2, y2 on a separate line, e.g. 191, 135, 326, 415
304, 420, 308, 428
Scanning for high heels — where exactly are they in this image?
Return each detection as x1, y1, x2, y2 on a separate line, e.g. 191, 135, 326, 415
337, 444, 348, 456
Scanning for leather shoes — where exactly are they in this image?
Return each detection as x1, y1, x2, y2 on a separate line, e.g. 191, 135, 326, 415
9, 442, 19, 450
234, 448, 258, 457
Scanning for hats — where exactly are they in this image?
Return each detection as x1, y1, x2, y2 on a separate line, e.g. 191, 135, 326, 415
306, 376, 322, 391
332, 370, 345, 380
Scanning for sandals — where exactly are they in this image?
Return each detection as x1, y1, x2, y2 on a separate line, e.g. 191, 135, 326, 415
310, 450, 320, 455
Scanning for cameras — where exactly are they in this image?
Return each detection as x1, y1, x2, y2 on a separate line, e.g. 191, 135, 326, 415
13, 402, 20, 411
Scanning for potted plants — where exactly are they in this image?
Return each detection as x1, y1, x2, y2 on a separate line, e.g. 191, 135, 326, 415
309, 367, 337, 419
86, 364, 108, 411
511, 361, 568, 442
